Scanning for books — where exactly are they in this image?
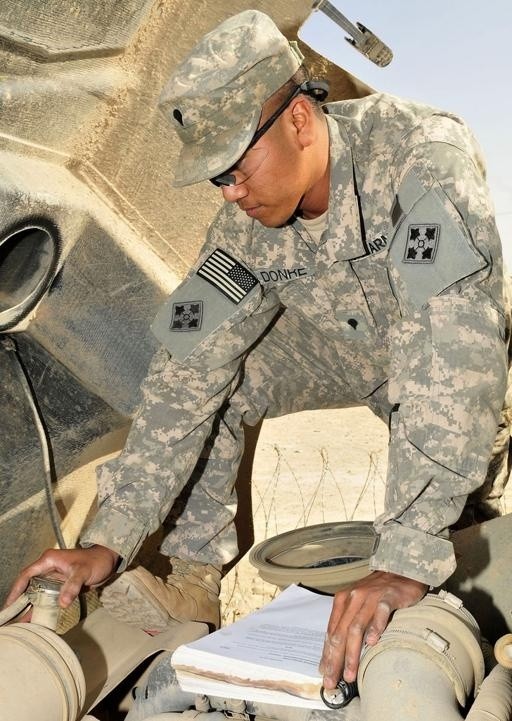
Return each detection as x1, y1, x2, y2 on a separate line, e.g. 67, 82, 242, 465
168, 579, 359, 712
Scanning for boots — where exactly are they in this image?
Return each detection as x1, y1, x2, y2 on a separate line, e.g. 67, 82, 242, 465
98, 555, 223, 638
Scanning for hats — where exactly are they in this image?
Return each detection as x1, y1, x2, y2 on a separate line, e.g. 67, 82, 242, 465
157, 9, 306, 188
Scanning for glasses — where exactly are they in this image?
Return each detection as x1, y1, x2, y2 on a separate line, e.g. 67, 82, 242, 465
209, 76, 308, 187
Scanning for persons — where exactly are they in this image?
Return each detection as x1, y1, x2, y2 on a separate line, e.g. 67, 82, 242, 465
2, 7, 512, 693
